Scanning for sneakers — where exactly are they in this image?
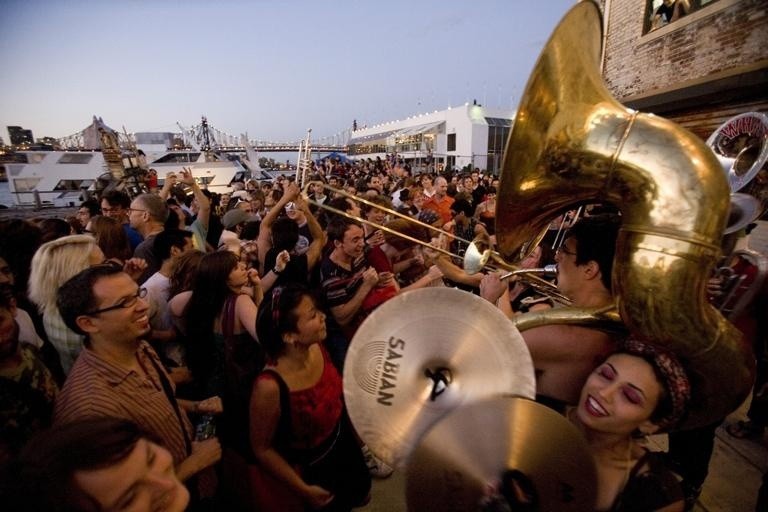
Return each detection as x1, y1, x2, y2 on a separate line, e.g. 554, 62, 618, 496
367, 457, 392, 478
726, 421, 764, 439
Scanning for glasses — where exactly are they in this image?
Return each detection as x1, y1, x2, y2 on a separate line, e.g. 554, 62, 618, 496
81, 288, 148, 315
125, 207, 145, 213
99, 206, 121, 213
556, 247, 576, 258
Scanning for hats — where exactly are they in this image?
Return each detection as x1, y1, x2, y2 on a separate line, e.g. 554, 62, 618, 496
221, 208, 258, 229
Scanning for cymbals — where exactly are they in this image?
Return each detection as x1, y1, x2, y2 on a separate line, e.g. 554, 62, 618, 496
343, 288, 539, 466
405, 394, 597, 511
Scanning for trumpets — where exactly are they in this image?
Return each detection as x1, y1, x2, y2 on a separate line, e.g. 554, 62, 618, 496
285, 128, 313, 210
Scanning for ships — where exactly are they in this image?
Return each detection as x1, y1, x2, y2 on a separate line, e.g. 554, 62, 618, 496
0, 117, 276, 209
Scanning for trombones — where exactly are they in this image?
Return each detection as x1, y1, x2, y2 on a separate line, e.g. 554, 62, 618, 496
302, 179, 575, 307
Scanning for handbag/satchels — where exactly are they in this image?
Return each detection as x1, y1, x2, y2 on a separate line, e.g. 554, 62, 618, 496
218, 360, 254, 398
217, 452, 301, 512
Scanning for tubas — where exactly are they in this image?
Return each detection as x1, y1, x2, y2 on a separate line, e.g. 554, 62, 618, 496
708, 193, 768, 324
706, 112, 768, 222
495, 0, 758, 428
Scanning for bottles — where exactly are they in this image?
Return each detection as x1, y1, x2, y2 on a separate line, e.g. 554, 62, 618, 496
196, 410, 216, 440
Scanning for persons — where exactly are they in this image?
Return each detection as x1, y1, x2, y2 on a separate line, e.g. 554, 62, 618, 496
647, 1, 701, 36
1, 152, 768, 512
